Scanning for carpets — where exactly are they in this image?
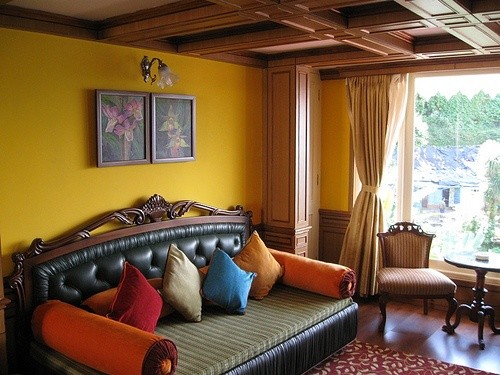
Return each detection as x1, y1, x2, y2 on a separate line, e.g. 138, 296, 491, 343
301, 340, 500, 375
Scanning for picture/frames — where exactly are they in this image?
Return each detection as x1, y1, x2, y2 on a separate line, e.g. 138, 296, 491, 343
93, 88, 150, 169
149, 91, 196, 164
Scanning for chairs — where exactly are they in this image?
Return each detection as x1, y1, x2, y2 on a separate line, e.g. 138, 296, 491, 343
375, 221, 458, 332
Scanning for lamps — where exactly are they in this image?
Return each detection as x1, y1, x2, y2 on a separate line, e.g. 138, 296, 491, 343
140, 55, 177, 88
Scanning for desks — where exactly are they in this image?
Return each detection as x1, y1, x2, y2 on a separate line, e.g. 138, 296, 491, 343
441, 250, 500, 351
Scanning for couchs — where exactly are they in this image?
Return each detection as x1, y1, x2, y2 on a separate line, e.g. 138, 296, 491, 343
3, 193, 361, 375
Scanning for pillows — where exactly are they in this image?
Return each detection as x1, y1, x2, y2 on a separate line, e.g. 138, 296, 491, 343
232, 229, 284, 300
164, 241, 202, 321
203, 247, 257, 317
80, 277, 176, 321
107, 263, 162, 334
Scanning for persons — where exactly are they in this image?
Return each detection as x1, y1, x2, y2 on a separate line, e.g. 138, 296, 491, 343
439, 197, 446, 213
